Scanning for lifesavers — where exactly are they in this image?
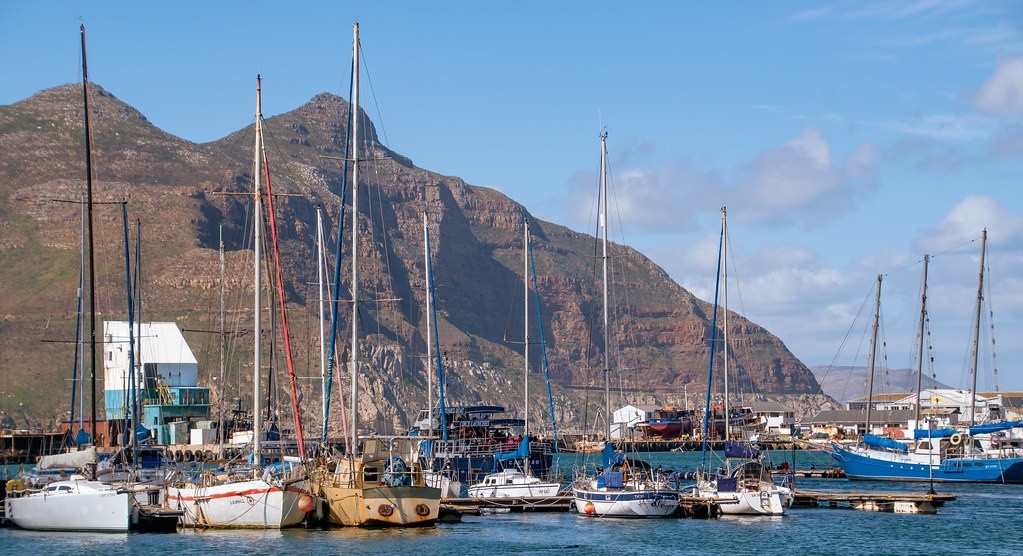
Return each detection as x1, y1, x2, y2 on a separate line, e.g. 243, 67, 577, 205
175, 449, 183, 462
218, 450, 227, 459
166, 451, 173, 464
194, 450, 202, 462
184, 449, 192, 462
461, 427, 473, 437
950, 433, 962, 445
990, 433, 1000, 446
616, 442, 724, 452
6, 479, 22, 492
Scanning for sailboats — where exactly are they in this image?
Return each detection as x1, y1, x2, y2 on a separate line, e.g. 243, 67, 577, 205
2, 19, 1023, 530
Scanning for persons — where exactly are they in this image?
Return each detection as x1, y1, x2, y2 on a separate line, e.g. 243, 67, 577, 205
458, 428, 539, 453
